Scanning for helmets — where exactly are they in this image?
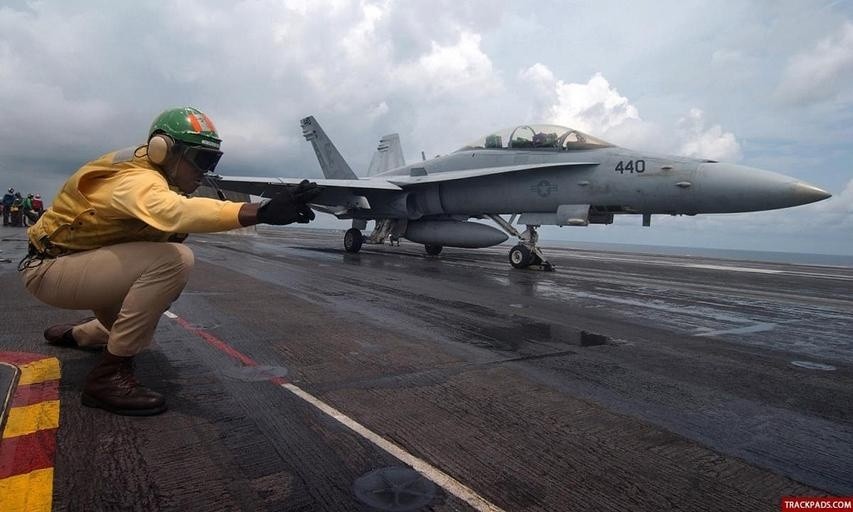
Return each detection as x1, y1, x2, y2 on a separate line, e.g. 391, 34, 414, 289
9, 187, 41, 198
147, 106, 222, 150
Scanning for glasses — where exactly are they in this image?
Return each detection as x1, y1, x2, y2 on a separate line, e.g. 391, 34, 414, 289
170, 142, 223, 172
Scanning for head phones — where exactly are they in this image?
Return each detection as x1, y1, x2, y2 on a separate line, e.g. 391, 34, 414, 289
134, 134, 174, 165
28, 195, 31, 197
11, 190, 13, 192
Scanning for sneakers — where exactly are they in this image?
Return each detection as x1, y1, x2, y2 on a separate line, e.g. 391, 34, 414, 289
3, 223, 30, 227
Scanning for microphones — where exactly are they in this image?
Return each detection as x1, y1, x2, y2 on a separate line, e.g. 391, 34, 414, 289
206, 175, 227, 201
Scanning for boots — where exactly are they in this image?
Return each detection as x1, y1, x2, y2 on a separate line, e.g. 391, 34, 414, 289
44, 316, 108, 352
80, 343, 169, 418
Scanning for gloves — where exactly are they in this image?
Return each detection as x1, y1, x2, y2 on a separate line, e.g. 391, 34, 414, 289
257, 180, 321, 226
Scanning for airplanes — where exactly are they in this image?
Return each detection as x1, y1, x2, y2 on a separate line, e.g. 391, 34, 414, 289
198, 114, 833, 273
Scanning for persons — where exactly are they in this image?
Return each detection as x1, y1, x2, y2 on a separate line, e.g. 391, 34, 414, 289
0, 186, 44, 227
17, 105, 325, 419
